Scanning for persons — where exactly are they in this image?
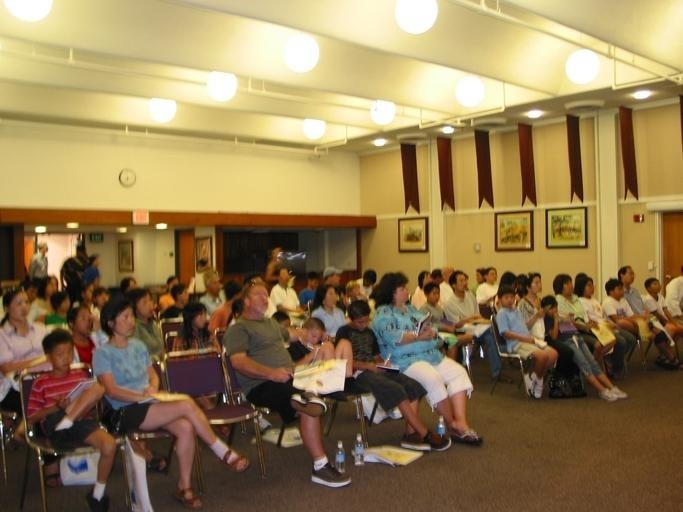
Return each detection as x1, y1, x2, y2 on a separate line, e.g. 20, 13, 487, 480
171, 300, 272, 435
163, 284, 188, 319
92, 296, 251, 508
541, 294, 629, 403
575, 273, 635, 378
419, 281, 473, 360
299, 271, 323, 305
410, 271, 441, 309
346, 279, 369, 304
369, 273, 484, 448
471, 268, 486, 293
618, 265, 681, 369
286, 317, 356, 398
553, 273, 615, 361
65, 301, 108, 371
496, 285, 558, 399
83, 253, 102, 285
207, 280, 241, 334
199, 270, 226, 315
73, 280, 101, 319
494, 272, 517, 312
28, 240, 49, 279
0, 277, 46, 324
188, 258, 208, 295
665, 266, 683, 317
336, 300, 453, 453
45, 291, 69, 325
266, 247, 296, 288
516, 274, 528, 301
310, 283, 347, 337
442, 271, 513, 382
644, 277, 683, 337
271, 312, 290, 329
120, 276, 136, 293
158, 276, 180, 310
224, 299, 244, 328
521, 272, 548, 343
431, 269, 443, 284
243, 273, 278, 318
28, 328, 117, 511
222, 281, 353, 488
358, 269, 377, 300
269, 266, 308, 325
59, 243, 87, 291
603, 279, 644, 335
91, 288, 111, 320
476, 267, 497, 305
311, 265, 346, 314
34, 275, 58, 311
127, 286, 166, 363
0, 284, 62, 487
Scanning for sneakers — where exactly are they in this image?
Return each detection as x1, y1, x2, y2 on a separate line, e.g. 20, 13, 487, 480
524, 371, 535, 397
655, 355, 682, 369
291, 392, 327, 418
598, 388, 617, 402
311, 462, 352, 488
258, 415, 272, 433
610, 386, 628, 399
400, 432, 431, 451
534, 376, 543, 399
85, 491, 110, 512
423, 429, 451, 452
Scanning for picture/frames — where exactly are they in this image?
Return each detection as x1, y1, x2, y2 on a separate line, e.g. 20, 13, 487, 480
545, 206, 589, 249
118, 238, 134, 272
194, 235, 213, 273
494, 210, 535, 252
398, 216, 428, 253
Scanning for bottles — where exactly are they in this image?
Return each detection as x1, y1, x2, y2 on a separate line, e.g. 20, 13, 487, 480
436, 415, 446, 439
354, 433, 364, 466
335, 439, 346, 473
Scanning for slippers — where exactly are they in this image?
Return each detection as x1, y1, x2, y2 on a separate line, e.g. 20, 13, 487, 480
455, 430, 483, 445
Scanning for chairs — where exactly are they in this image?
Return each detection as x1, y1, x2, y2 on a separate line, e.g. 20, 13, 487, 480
164, 347, 267, 495
164, 331, 178, 353
214, 326, 228, 353
159, 311, 215, 346
323, 337, 407, 444
19, 365, 135, 512
220, 353, 306, 447
0, 408, 20, 485
490, 313, 555, 400
91, 355, 192, 507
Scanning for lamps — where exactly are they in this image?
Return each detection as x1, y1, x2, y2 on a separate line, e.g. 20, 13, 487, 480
443, 126, 454, 134
151, 99, 175, 122
526, 109, 540, 118
6, 2, 53, 21
371, 101, 398, 125
286, 33, 318, 71
207, 70, 235, 100
457, 76, 485, 106
566, 50, 598, 84
635, 90, 651, 100
302, 118, 324, 137
373, 138, 385, 146
395, 0, 437, 34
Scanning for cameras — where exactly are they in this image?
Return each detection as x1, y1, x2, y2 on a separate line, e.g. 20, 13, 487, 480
276, 251, 306, 266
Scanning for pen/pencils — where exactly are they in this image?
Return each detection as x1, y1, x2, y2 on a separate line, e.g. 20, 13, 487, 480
383, 353, 392, 367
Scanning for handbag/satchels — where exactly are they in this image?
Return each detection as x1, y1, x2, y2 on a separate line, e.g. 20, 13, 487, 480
548, 377, 588, 399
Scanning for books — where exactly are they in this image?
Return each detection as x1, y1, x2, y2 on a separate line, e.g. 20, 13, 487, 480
292, 358, 345, 394
365, 444, 423, 466
417, 311, 432, 334
65, 380, 94, 400
376, 363, 401, 372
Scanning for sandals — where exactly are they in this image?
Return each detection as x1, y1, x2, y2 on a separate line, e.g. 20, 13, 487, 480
146, 458, 169, 474
218, 449, 251, 473
175, 482, 203, 510
41, 453, 63, 489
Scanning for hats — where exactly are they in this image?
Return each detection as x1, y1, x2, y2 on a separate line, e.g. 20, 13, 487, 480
322, 266, 343, 279
